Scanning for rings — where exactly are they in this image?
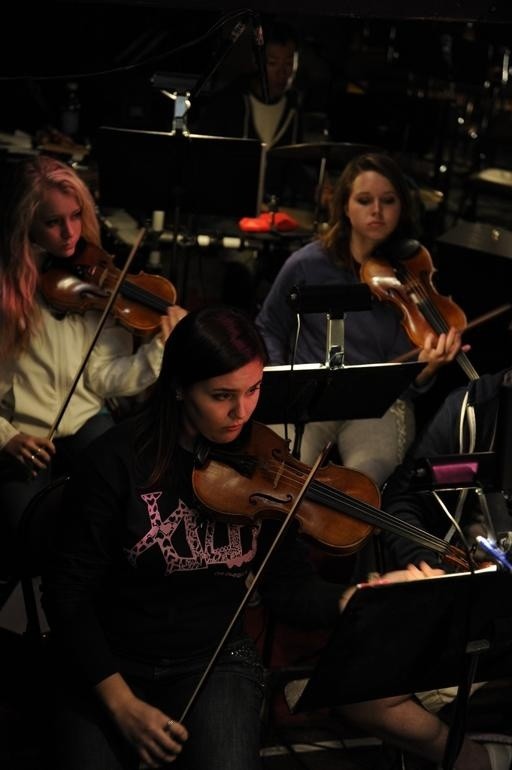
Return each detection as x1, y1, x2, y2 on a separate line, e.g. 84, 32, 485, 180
164, 718, 175, 731
34, 447, 44, 456
27, 454, 36, 463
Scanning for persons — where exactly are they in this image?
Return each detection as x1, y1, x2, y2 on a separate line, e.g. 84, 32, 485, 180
1, 151, 190, 478
251, 145, 467, 495
221, 21, 310, 166
58, 305, 511, 768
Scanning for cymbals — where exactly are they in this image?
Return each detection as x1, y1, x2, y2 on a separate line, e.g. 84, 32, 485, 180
266, 141, 371, 158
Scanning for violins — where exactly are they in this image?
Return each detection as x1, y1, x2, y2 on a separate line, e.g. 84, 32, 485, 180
191, 418, 479, 573
357, 240, 479, 389
38, 235, 180, 340
